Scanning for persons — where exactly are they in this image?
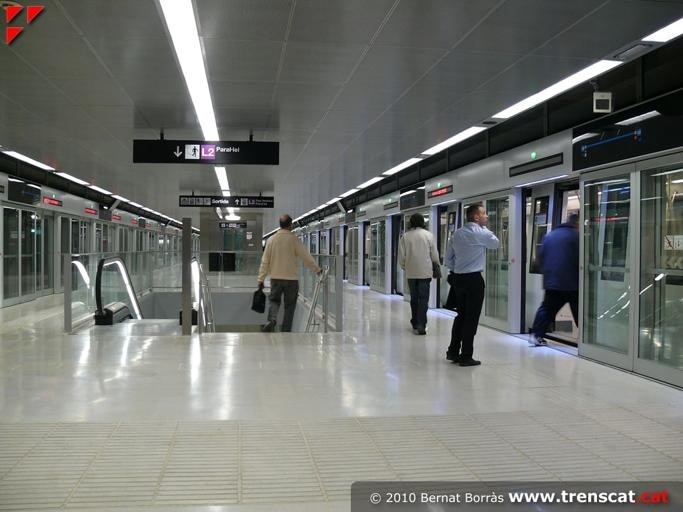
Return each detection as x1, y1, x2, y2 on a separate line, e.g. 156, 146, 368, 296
398, 212, 443, 335
444, 202, 501, 367
527, 213, 579, 346
257, 214, 322, 332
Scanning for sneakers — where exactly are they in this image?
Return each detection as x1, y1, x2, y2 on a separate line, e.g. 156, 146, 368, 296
410, 318, 427, 335
444, 351, 481, 367
528, 335, 547, 347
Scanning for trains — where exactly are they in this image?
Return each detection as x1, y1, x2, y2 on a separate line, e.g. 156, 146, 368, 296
291, 88, 683, 391
0, 172, 200, 308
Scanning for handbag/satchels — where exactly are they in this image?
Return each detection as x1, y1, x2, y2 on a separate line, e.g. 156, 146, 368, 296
250, 286, 266, 313
432, 262, 442, 279
